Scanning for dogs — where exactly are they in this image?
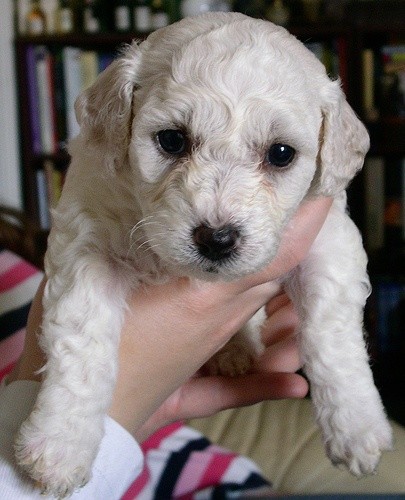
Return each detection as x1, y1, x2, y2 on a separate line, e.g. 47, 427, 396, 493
11, 8, 394, 500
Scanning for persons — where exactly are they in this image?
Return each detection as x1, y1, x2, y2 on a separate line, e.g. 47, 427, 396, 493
0, 191, 336, 500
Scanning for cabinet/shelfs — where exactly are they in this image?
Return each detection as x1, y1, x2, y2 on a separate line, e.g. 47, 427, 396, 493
11, 0, 404, 427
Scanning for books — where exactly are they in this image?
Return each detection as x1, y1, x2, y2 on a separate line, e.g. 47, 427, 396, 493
16, 1, 405, 248
